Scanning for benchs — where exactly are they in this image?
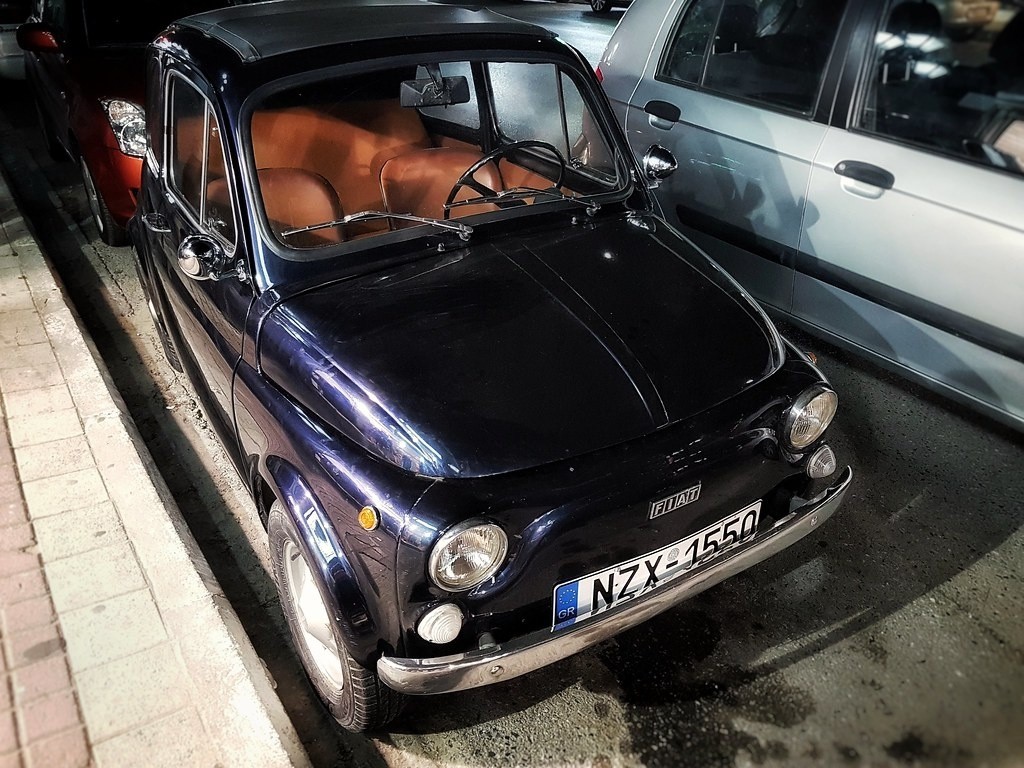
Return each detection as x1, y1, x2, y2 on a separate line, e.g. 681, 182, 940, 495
174, 98, 433, 237
671, 0, 833, 114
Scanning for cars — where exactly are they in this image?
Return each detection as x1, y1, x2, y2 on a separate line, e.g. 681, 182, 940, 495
15, 0, 251, 248
128, 0, 858, 734
578, 0, 1023, 432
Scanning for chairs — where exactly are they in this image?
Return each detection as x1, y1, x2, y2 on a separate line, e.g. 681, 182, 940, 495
195, 167, 346, 265
378, 149, 503, 230
